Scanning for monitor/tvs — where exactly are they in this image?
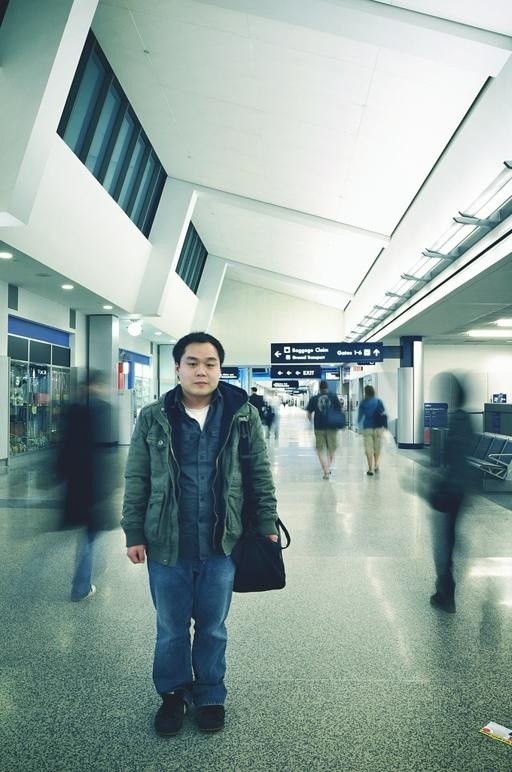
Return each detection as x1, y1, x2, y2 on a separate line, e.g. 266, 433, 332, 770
492, 393, 506, 403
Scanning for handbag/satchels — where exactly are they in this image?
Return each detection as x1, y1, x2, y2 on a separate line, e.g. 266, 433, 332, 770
324, 409, 345, 427
232, 516, 289, 593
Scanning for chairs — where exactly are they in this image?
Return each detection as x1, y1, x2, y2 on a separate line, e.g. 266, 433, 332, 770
464, 431, 512, 480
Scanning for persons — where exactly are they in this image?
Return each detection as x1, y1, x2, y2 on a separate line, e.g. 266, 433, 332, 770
306, 380, 341, 480
120, 331, 279, 737
249, 387, 263, 421
357, 385, 385, 476
415, 371, 473, 614
58, 370, 118, 603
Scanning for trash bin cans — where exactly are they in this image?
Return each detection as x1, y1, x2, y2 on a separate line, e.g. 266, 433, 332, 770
430, 428, 450, 466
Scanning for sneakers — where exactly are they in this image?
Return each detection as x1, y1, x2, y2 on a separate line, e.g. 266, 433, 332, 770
153, 692, 187, 737
428, 591, 454, 615
75, 583, 96, 602
194, 700, 226, 730
321, 467, 381, 481
